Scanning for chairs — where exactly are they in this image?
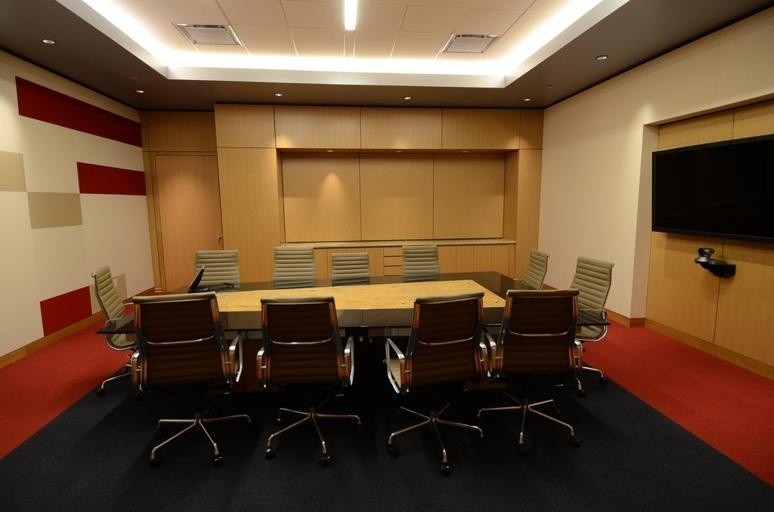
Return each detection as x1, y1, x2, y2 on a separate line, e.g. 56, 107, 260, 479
332, 254, 370, 279
478, 289, 582, 451
518, 250, 550, 290
383, 292, 491, 475
568, 257, 615, 396
400, 244, 440, 277
132, 291, 253, 468
90, 266, 138, 397
256, 297, 363, 467
192, 249, 239, 287
271, 248, 316, 280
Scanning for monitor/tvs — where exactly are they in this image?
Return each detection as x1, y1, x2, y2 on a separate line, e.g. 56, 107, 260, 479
187, 265, 208, 292
651, 134, 774, 243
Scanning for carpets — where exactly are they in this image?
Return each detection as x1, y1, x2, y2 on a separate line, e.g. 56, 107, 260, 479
0, 352, 774, 509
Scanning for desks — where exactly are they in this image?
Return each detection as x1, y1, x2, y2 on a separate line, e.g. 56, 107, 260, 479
94, 271, 611, 396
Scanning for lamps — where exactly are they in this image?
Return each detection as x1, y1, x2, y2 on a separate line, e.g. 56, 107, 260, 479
344, 0, 357, 31
